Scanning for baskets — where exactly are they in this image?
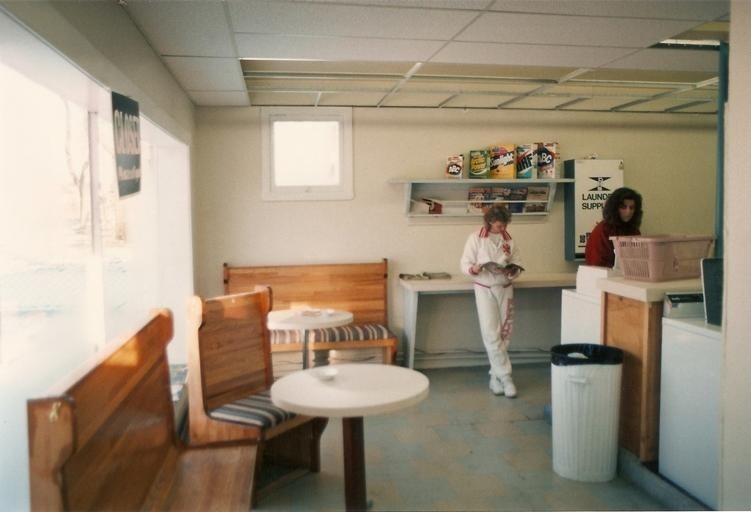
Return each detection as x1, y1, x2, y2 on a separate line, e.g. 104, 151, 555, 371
609, 231, 717, 282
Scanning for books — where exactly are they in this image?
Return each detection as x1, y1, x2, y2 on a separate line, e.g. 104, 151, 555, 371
423, 271, 452, 279
398, 272, 430, 281
480, 261, 526, 274
466, 187, 549, 214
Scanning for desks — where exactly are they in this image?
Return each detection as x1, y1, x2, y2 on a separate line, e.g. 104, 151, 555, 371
265, 308, 430, 511
398, 272, 576, 368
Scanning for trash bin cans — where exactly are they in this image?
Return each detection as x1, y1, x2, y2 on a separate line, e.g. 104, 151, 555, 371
551, 344, 623, 483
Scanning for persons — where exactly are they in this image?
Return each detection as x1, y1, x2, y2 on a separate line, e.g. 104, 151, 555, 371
460, 204, 525, 398
586, 186, 644, 268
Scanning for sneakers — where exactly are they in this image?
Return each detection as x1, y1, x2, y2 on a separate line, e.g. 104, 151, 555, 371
490, 375, 504, 394
504, 377, 517, 397
